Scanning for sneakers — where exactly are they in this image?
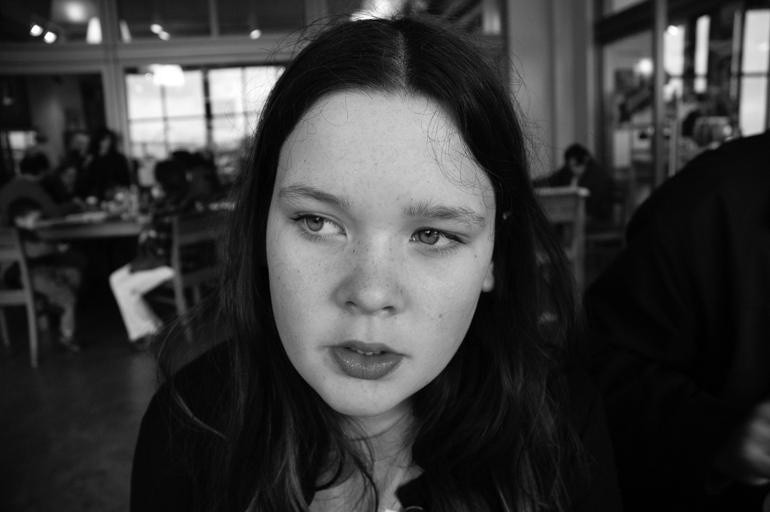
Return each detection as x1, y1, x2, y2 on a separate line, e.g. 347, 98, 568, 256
60, 325, 167, 355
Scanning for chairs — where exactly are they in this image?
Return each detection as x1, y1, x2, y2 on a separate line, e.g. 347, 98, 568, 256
531, 186, 592, 322
1, 227, 57, 370
147, 216, 224, 345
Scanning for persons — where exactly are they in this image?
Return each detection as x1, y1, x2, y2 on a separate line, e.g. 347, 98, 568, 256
129, 15, 620, 510
0, 123, 210, 365
564, 142, 619, 232
